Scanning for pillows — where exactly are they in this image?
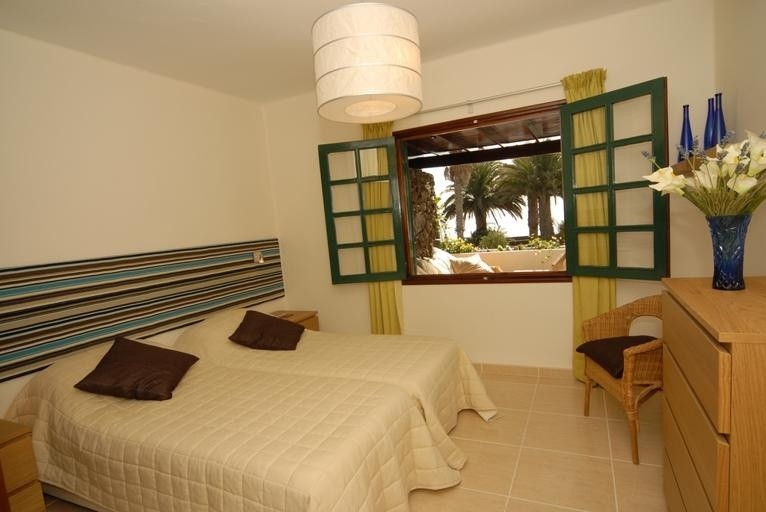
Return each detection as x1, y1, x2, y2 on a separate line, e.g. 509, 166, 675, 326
415, 246, 495, 275
576, 335, 659, 380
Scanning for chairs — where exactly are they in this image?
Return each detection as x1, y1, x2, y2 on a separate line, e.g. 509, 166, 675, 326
581, 294, 663, 465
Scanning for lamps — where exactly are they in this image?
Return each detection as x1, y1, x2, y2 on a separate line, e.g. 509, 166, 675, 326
311, 3, 423, 124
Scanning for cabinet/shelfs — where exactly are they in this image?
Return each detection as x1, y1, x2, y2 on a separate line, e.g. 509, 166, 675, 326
660, 275, 766, 512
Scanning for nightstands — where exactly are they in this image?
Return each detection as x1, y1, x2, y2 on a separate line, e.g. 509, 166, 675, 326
0, 417, 46, 512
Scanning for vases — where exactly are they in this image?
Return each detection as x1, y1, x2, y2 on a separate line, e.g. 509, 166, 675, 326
705, 216, 753, 290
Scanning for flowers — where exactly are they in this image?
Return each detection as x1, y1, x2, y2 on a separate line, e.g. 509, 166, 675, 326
640, 127, 766, 217
